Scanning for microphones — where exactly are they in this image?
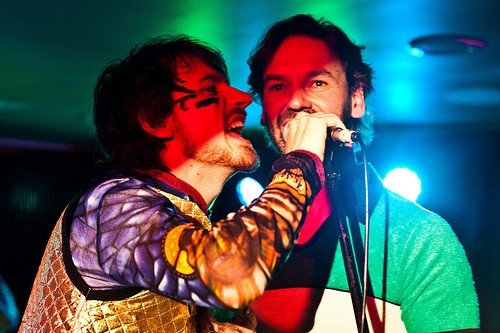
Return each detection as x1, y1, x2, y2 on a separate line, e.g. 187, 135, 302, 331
326, 128, 359, 144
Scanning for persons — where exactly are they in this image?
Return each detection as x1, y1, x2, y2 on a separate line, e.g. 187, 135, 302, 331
16, 32, 354, 333
208, 14, 483, 333
